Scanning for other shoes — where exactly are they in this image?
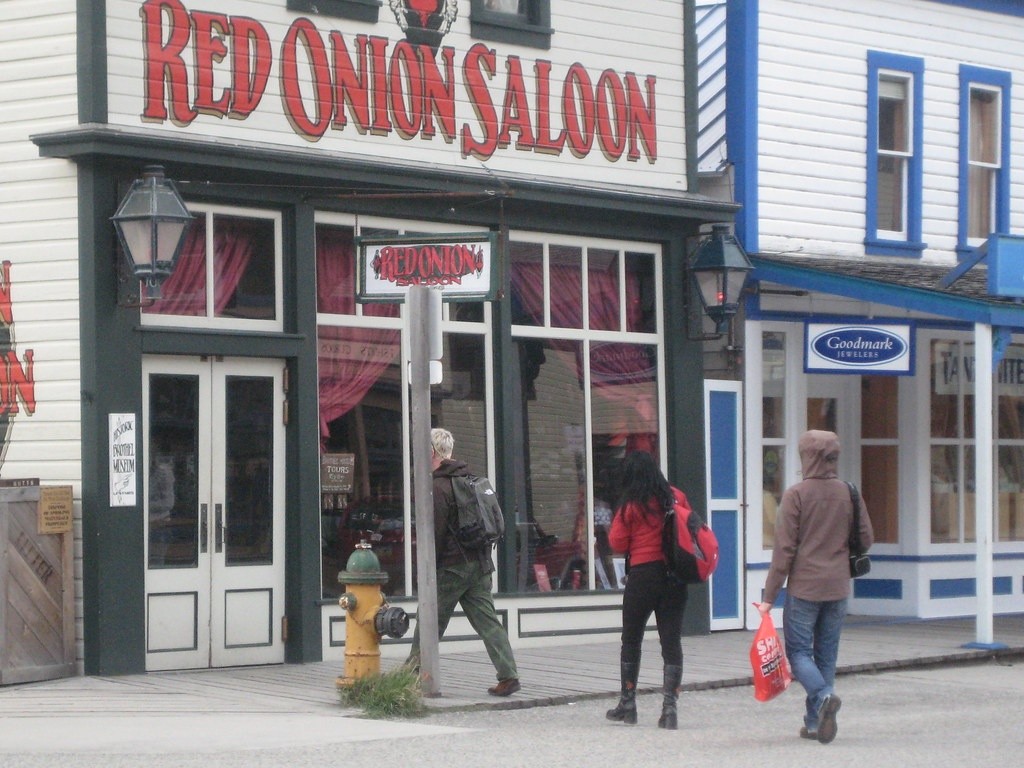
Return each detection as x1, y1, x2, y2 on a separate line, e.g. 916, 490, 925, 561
817, 694, 841, 745
798, 727, 820, 741
487, 678, 521, 697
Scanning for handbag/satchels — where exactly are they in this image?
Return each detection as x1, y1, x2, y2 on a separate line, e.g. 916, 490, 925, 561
750, 602, 792, 701
849, 554, 871, 578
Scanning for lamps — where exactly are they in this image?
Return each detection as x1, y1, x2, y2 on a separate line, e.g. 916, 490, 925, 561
107, 162, 197, 308
686, 223, 757, 341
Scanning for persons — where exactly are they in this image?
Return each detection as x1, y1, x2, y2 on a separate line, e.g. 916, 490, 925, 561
609, 449, 691, 730
149, 426, 175, 569
758, 429, 874, 744
402, 428, 521, 696
556, 482, 614, 591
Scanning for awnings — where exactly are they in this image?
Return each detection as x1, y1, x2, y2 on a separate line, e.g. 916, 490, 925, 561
749, 253, 1024, 645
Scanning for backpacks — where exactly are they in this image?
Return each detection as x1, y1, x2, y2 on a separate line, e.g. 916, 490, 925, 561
664, 502, 719, 583
435, 471, 502, 540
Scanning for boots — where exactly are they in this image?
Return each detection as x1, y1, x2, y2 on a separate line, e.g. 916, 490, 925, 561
606, 661, 642, 725
657, 663, 683, 729
